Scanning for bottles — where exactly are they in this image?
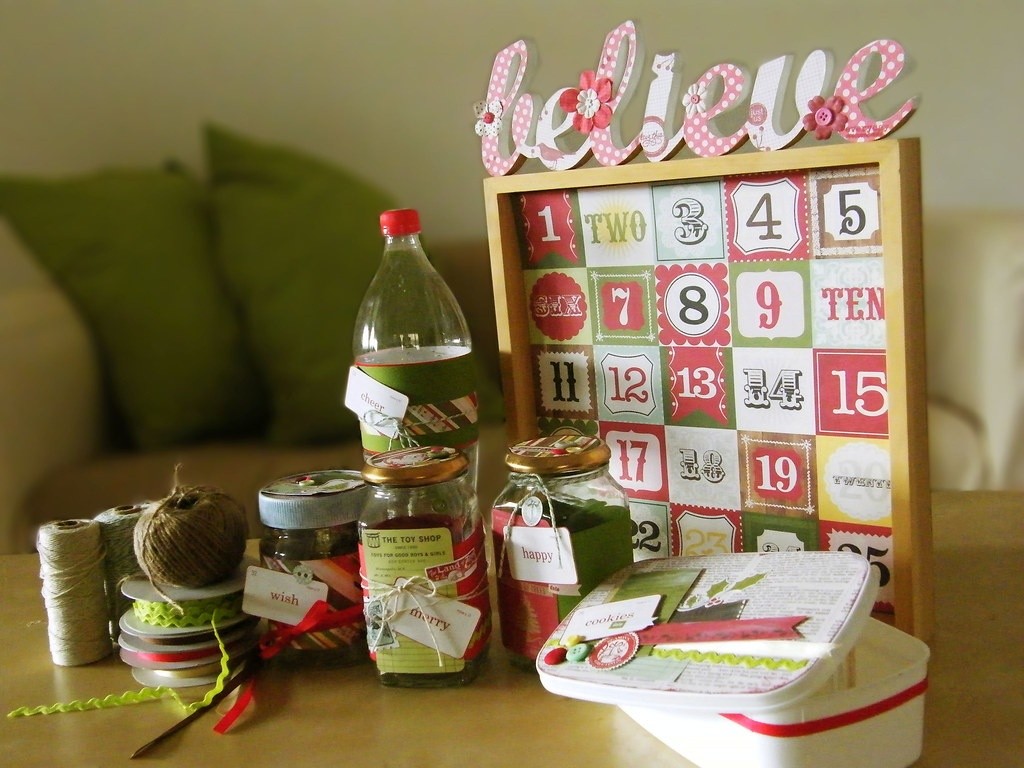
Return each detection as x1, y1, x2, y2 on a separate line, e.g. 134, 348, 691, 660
491, 433, 635, 677
351, 208, 480, 497
356, 445, 492, 691
257, 469, 371, 670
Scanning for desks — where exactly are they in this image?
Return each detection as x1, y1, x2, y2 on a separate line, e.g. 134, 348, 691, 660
0, 491, 1024, 768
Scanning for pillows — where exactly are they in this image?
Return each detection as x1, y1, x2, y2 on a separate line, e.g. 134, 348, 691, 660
0, 155, 255, 452
201, 119, 432, 446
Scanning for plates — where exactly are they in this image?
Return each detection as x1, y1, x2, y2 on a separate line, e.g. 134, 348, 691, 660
118, 555, 259, 689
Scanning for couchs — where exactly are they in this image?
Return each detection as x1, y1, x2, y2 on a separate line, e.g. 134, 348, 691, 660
0, 205, 1024, 553
